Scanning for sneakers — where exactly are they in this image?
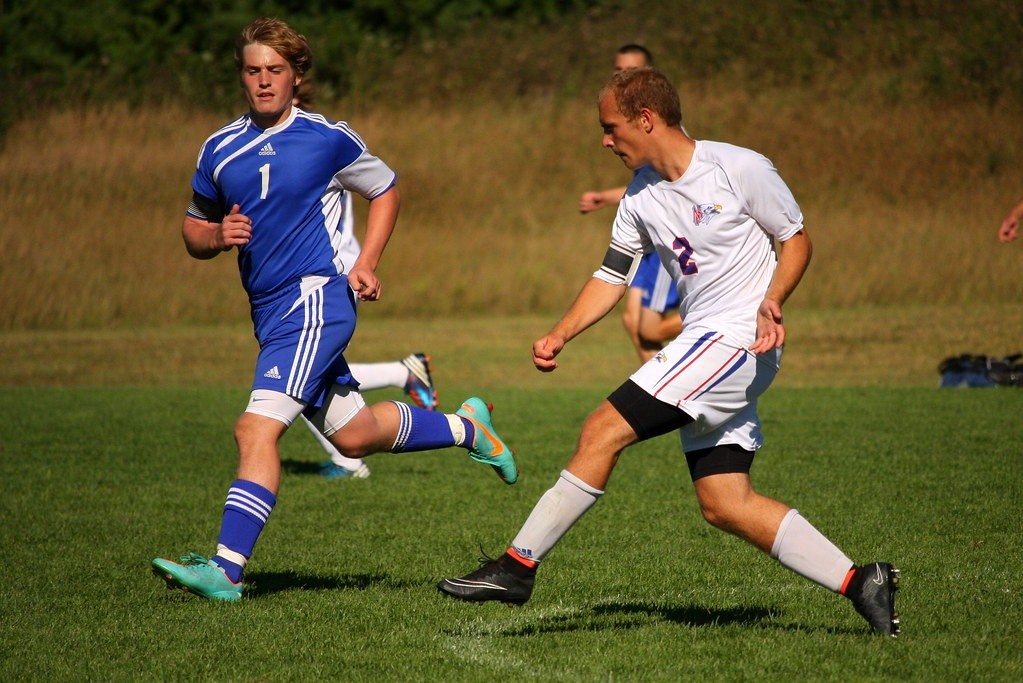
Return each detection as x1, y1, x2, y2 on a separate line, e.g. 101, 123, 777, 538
150, 552, 244, 605
455, 396, 521, 486
312, 455, 372, 480
400, 353, 439, 413
436, 541, 536, 606
852, 562, 902, 638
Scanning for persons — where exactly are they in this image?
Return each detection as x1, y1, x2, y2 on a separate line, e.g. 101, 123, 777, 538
439, 66, 900, 645
152, 18, 519, 600
283, 68, 437, 483
581, 44, 682, 360
